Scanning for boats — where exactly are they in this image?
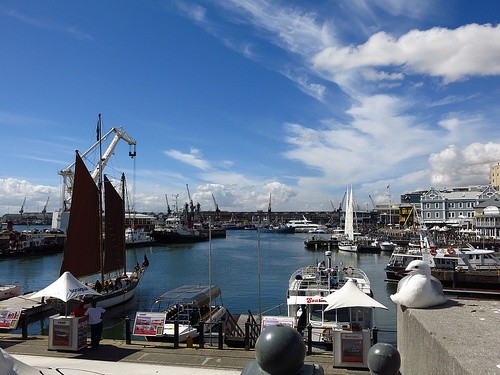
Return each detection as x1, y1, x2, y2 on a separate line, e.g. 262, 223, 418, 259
257, 216, 295, 234
383, 237, 500, 296
269, 219, 330, 234
260, 250, 376, 352
370, 234, 398, 251
302, 228, 342, 248
147, 209, 209, 243
142, 283, 229, 344
222, 223, 245, 230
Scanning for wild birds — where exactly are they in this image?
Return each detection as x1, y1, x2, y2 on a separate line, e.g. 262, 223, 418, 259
389, 259, 448, 309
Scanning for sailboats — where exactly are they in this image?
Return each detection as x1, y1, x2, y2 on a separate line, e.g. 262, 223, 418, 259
337, 183, 383, 254
46, 114, 151, 316
392, 204, 443, 254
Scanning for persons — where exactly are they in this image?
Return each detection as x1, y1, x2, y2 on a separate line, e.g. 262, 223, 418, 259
297, 309, 306, 337
189, 310, 199, 325
84, 301, 106, 351
95, 280, 102, 294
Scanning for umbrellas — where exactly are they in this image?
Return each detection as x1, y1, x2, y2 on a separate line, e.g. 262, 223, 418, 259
323, 279, 388, 331
27, 271, 102, 320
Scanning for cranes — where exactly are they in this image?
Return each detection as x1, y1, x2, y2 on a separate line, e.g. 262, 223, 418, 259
50, 125, 137, 229
